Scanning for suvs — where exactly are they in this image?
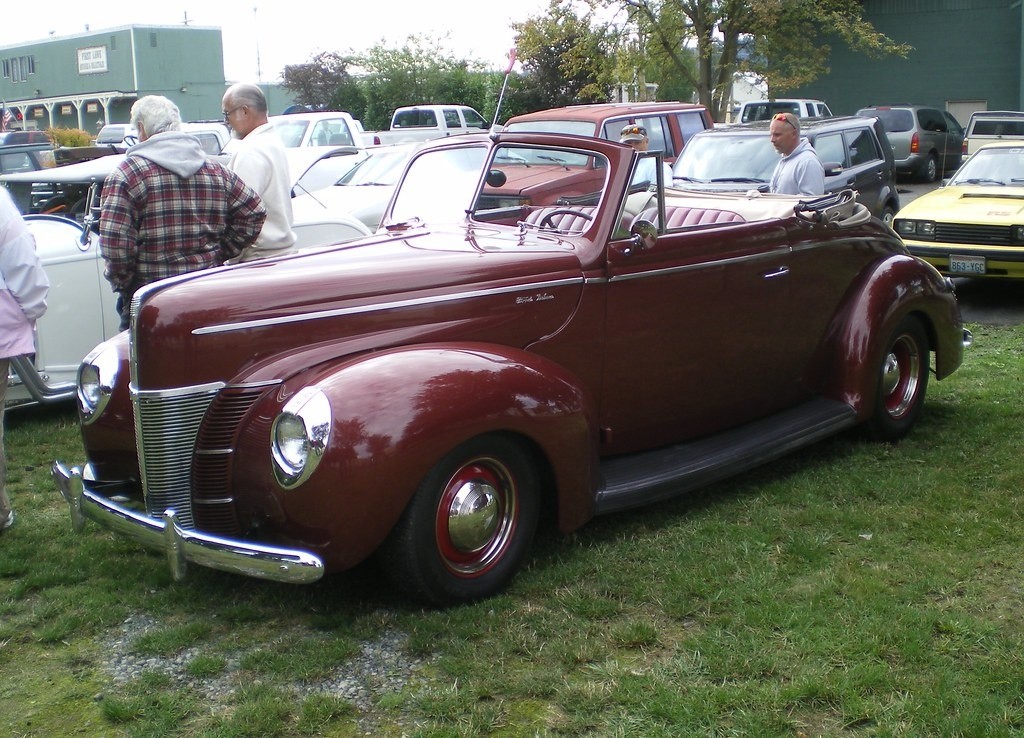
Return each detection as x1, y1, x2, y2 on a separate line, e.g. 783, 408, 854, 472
668, 114, 899, 227
854, 102, 964, 183
960, 110, 1024, 166
734, 99, 834, 125
475, 101, 715, 228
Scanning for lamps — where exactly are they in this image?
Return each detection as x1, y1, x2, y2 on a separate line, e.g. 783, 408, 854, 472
180, 87, 188, 93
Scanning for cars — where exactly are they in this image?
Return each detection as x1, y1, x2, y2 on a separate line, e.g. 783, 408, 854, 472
291, 147, 434, 232
49, 128, 970, 611
0, 120, 231, 234
891, 143, 1024, 286
0, 148, 374, 411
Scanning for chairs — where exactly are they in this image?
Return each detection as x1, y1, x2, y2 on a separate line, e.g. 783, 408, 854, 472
628, 206, 746, 232
524, 206, 639, 235
329, 134, 347, 145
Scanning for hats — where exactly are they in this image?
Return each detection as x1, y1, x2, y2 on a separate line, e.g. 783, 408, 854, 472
619, 124, 647, 142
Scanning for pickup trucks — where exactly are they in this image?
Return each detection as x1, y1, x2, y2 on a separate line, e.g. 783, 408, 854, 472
265, 112, 389, 159
356, 105, 503, 145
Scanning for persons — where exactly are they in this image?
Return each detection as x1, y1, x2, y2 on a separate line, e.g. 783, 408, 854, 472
222, 82, 297, 260
769, 112, 825, 196
0, 182, 51, 530
620, 123, 674, 188
317, 121, 333, 146
99, 95, 267, 331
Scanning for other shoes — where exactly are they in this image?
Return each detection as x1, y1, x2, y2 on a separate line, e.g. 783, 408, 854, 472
0, 510, 14, 529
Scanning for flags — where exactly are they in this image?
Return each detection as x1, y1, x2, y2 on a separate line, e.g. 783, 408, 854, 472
1, 102, 13, 132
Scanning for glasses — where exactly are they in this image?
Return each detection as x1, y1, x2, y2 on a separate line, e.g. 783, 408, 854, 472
222, 106, 241, 118
773, 114, 797, 130
620, 128, 640, 135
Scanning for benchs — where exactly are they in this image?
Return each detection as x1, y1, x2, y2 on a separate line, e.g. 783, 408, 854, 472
624, 191, 821, 221
